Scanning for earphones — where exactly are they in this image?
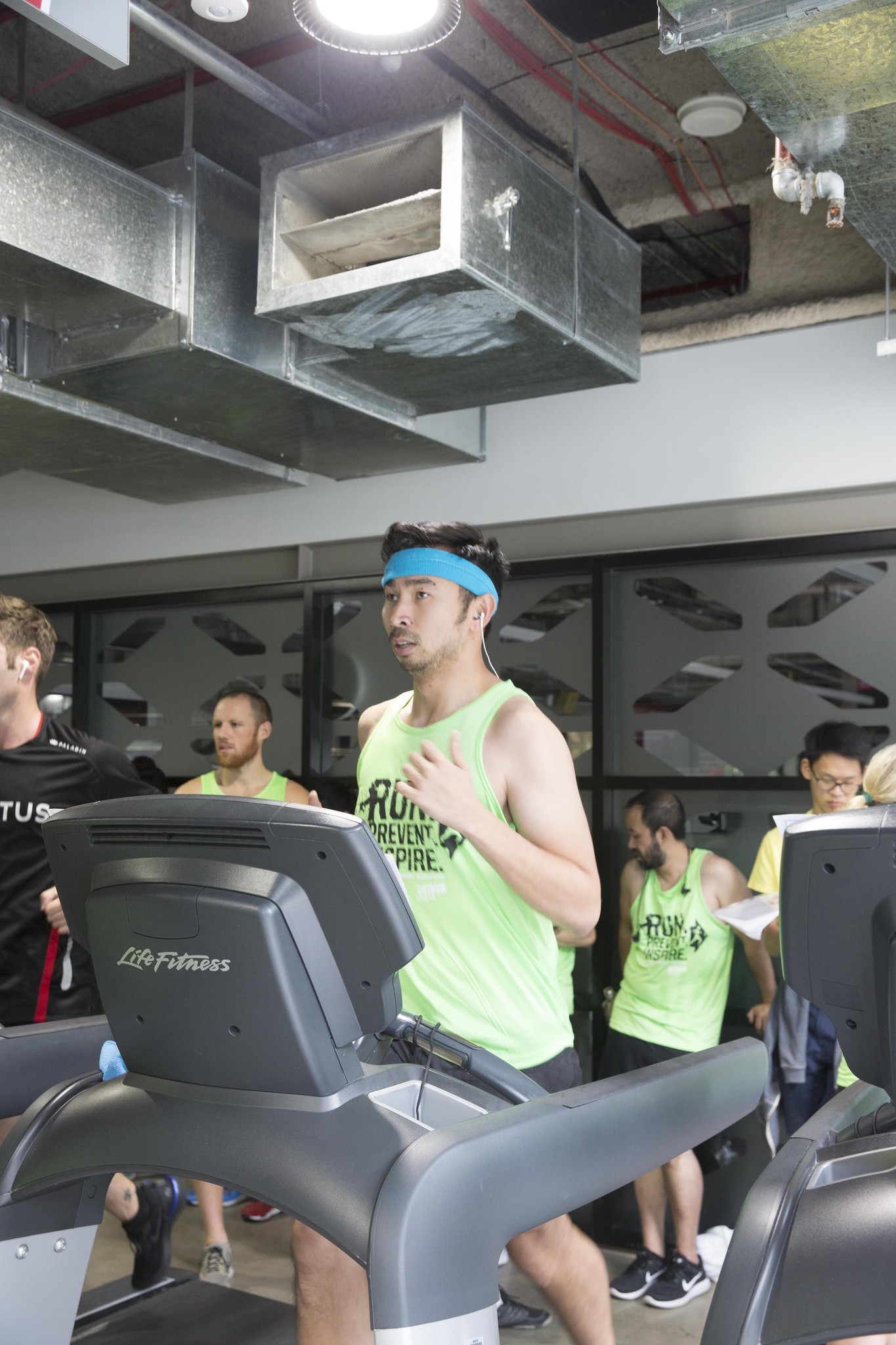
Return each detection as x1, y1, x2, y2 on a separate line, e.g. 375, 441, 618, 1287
475, 611, 485, 629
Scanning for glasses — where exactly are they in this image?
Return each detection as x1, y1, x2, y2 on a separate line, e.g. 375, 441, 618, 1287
810, 762, 858, 796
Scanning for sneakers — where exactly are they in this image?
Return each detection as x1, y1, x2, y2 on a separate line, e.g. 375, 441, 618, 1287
185, 1190, 198, 1206
610, 1243, 672, 1300
495, 1284, 553, 1329
199, 1242, 235, 1289
119, 1174, 178, 1291
644, 1251, 711, 1309
241, 1198, 281, 1222
223, 1185, 248, 1207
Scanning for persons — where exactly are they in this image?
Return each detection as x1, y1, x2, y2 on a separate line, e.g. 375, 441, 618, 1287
0, 595, 310, 1292
599, 787, 777, 1308
309, 519, 616, 1345
833, 743, 896, 1093
747, 722, 873, 1139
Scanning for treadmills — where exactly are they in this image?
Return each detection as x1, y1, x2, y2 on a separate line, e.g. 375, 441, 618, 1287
698, 807, 894, 1345
1, 795, 768, 1345
